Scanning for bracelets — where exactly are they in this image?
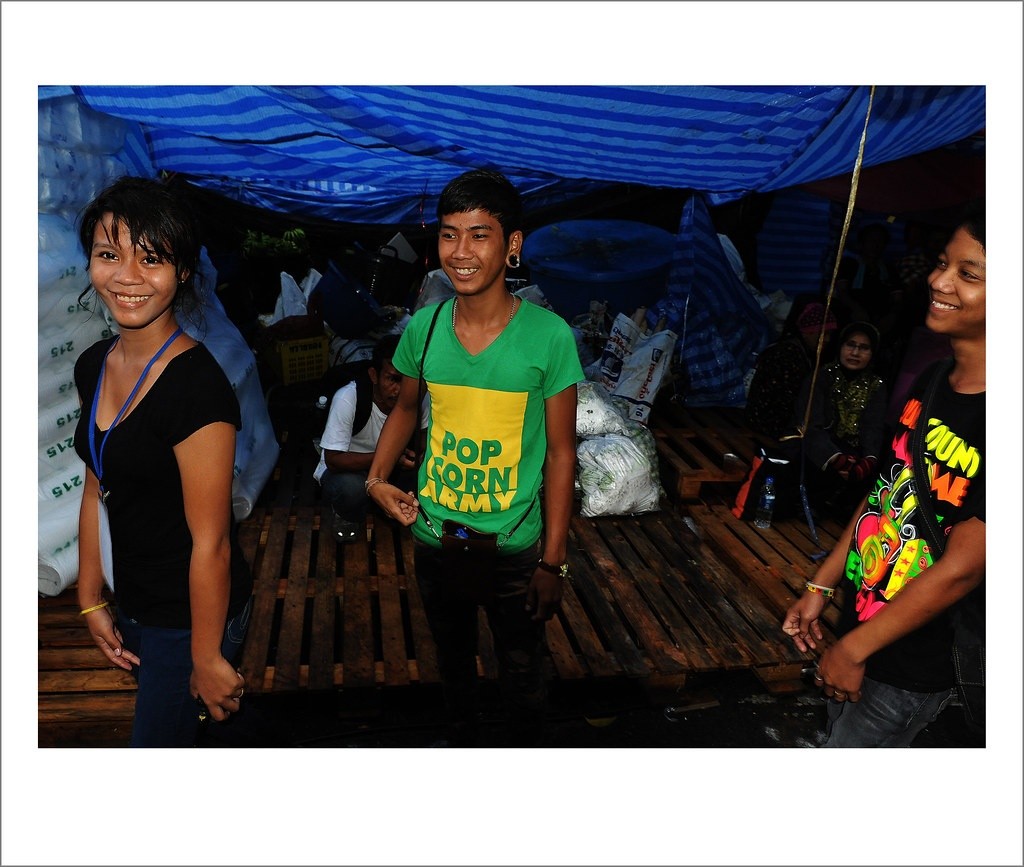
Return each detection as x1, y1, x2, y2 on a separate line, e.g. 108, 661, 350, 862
806, 580, 835, 599
80, 602, 109, 615
365, 478, 388, 497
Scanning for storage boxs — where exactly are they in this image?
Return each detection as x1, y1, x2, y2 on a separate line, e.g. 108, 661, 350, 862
257, 310, 336, 389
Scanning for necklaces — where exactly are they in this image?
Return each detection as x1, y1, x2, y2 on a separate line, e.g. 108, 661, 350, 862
453, 293, 515, 329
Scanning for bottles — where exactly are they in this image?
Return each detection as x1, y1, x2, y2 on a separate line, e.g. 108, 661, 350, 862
316, 396, 329, 410
753, 478, 776, 529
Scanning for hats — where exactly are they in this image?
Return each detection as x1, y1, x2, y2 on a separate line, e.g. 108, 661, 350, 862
796, 303, 837, 333
839, 321, 881, 352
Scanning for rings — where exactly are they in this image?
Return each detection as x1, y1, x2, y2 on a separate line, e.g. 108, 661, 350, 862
834, 691, 845, 696
815, 674, 824, 681
238, 687, 244, 698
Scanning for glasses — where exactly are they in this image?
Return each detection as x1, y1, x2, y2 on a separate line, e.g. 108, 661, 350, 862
841, 340, 871, 355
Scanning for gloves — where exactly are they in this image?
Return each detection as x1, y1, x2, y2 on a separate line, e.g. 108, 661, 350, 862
833, 454, 856, 474
855, 456, 879, 481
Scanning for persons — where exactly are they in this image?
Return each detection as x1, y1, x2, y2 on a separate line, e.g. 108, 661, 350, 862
782, 216, 986, 749
312, 334, 431, 544
74, 177, 271, 748
363, 172, 589, 749
787, 323, 888, 511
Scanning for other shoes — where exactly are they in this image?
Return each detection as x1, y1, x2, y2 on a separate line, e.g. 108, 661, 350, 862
331, 508, 360, 544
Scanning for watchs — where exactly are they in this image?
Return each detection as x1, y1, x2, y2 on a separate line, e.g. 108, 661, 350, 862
538, 558, 568, 578
